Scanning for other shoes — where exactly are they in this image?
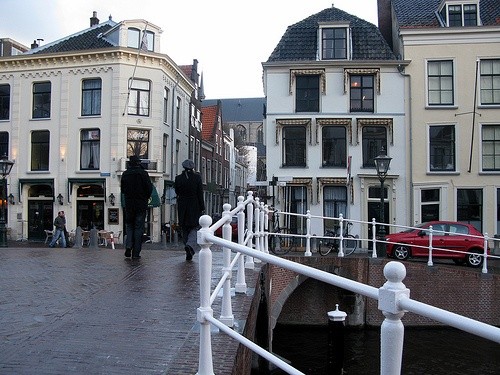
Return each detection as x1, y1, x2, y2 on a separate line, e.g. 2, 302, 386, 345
125, 248, 131, 257
132, 254, 141, 258
185, 246, 192, 261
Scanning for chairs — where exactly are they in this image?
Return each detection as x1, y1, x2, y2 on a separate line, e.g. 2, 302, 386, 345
44, 229, 122, 248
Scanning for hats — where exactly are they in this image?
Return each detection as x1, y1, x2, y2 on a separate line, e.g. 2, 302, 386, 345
182, 160, 194, 169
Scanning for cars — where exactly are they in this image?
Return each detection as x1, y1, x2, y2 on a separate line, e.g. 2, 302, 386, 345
384, 221, 491, 268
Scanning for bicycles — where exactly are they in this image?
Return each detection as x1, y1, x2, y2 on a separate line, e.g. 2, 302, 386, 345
319, 221, 357, 256
266, 210, 294, 255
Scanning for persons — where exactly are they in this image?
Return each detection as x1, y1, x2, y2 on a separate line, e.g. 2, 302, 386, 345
121, 154, 160, 258
174, 160, 205, 263
48, 211, 73, 248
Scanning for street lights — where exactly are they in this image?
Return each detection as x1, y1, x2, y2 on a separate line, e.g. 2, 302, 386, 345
374, 146, 392, 256
0, 152, 15, 247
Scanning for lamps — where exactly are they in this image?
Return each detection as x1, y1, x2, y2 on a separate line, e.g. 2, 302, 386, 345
109, 193, 116, 206
57, 193, 64, 205
8, 193, 16, 205
